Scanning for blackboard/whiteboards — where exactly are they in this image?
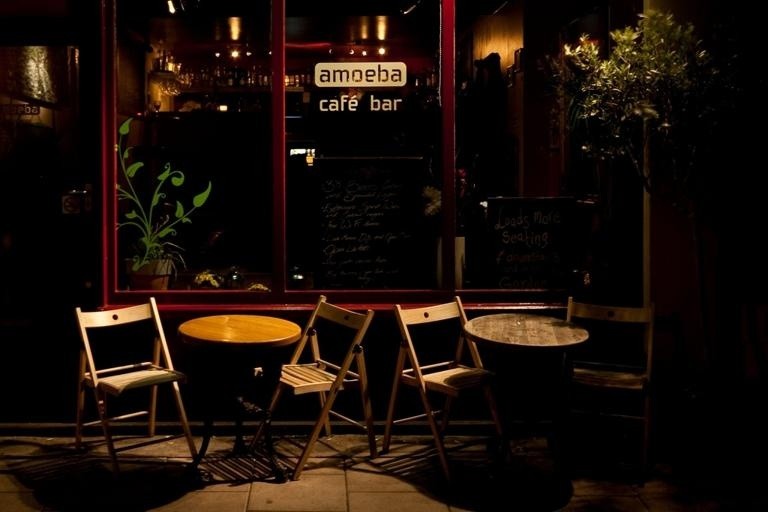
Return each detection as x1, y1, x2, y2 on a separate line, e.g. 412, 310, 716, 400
305, 157, 598, 289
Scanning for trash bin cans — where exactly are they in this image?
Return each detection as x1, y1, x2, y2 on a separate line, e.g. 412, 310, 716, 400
511, 436, 555, 511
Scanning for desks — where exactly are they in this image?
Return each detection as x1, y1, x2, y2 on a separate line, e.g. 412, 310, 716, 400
460, 312, 590, 451
174, 310, 304, 485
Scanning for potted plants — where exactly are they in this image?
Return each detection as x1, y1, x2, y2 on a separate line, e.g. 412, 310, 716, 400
113, 119, 217, 293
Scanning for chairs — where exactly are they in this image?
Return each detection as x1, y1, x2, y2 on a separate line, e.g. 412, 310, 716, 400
564, 296, 658, 449
71, 299, 201, 479
239, 294, 378, 482
382, 296, 503, 479
